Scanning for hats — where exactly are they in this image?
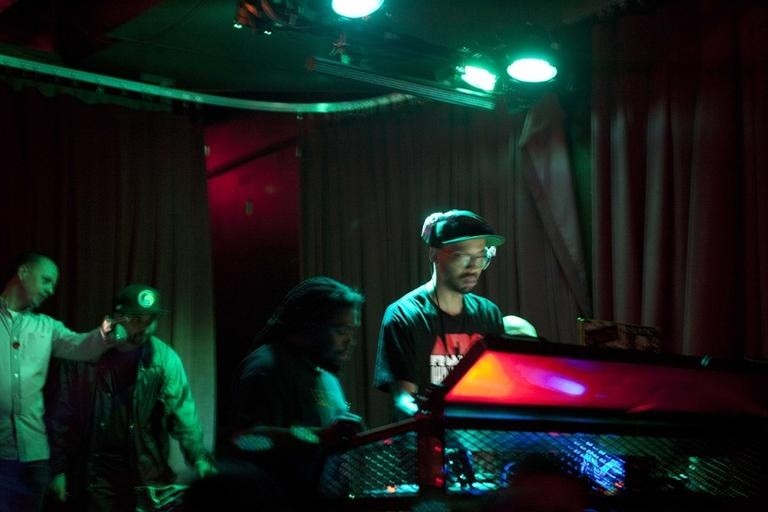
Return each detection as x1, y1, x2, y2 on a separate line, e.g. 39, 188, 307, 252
429, 207, 506, 252
114, 282, 170, 318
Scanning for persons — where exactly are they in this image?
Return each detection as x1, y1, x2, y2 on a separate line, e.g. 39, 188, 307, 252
223, 269, 403, 496
45, 282, 215, 511
365, 200, 509, 469
2, 252, 120, 510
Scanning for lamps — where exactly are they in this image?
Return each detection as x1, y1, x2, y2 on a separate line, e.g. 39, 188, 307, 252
460, 10, 560, 94
